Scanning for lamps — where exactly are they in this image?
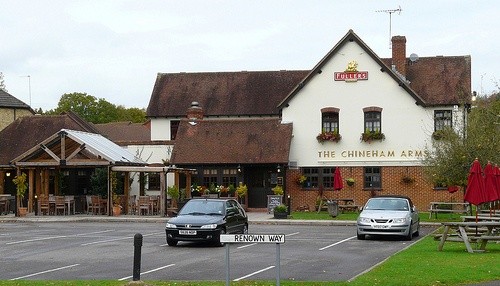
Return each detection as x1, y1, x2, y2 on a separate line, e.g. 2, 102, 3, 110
237, 163, 241, 171
277, 163, 281, 172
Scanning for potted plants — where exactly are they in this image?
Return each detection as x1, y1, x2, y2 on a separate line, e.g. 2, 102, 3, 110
13, 172, 28, 217
296, 174, 307, 185
346, 178, 355, 186
274, 205, 287, 219
166, 185, 179, 217
237, 184, 248, 210
113, 192, 120, 215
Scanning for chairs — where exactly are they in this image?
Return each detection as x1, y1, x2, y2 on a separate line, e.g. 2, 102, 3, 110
49, 194, 160, 216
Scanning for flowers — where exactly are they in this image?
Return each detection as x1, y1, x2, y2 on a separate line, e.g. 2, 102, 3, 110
191, 182, 235, 194
317, 127, 341, 145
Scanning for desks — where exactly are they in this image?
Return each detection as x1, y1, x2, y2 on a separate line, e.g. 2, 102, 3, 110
38, 200, 75, 216
439, 210, 500, 253
315, 198, 358, 214
429, 202, 469, 220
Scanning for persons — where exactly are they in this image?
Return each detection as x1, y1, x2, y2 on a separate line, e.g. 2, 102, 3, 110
191, 184, 201, 199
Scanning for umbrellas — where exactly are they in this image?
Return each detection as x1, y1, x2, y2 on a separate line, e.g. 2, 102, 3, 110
333, 166, 343, 203
464, 158, 489, 237
491, 163, 500, 214
448, 185, 458, 210
482, 160, 499, 217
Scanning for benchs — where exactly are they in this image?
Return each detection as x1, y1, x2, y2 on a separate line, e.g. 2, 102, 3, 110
429, 209, 469, 212
435, 233, 485, 237
322, 205, 358, 208
481, 236, 500, 239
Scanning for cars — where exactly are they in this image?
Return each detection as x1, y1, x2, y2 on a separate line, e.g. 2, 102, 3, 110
356, 194, 420, 241
165, 197, 249, 247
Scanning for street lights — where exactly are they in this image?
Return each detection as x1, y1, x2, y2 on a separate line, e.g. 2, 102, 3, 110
27, 75, 31, 106
